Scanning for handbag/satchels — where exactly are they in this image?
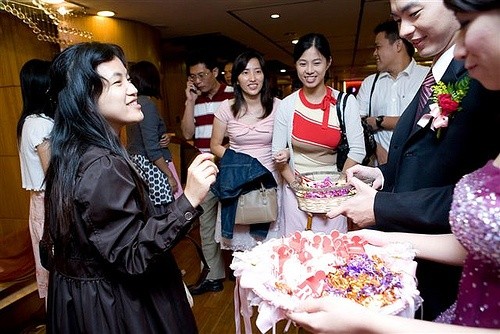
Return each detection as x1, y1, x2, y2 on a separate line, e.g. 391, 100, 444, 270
234, 182, 278, 225
336, 90, 377, 172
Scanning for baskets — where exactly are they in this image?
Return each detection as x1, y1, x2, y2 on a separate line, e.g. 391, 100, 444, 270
289, 172, 375, 214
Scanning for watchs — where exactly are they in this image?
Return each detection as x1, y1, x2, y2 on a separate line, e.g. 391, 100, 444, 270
376, 115, 385, 131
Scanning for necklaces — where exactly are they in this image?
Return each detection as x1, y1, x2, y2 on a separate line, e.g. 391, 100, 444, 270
240, 108, 263, 115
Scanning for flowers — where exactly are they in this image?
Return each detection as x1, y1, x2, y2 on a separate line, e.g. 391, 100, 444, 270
430, 76, 471, 139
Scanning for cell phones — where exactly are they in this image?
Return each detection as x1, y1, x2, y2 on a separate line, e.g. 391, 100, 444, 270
190, 83, 199, 93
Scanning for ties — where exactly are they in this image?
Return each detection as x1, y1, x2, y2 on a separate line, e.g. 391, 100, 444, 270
412, 71, 437, 130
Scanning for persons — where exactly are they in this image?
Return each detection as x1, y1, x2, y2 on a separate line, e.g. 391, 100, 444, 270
221, 63, 233, 85
125, 60, 183, 207
278, 0, 500, 334
39, 41, 219, 334
322, 0, 500, 321
210, 50, 291, 251
272, 33, 377, 235
180, 54, 236, 295
356, 21, 431, 230
16, 59, 56, 311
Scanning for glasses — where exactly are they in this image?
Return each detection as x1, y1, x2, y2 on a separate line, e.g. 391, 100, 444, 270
221, 70, 232, 75
186, 72, 212, 81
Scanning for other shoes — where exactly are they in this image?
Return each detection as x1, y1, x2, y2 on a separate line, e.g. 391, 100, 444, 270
230, 269, 237, 280
190, 279, 223, 295
